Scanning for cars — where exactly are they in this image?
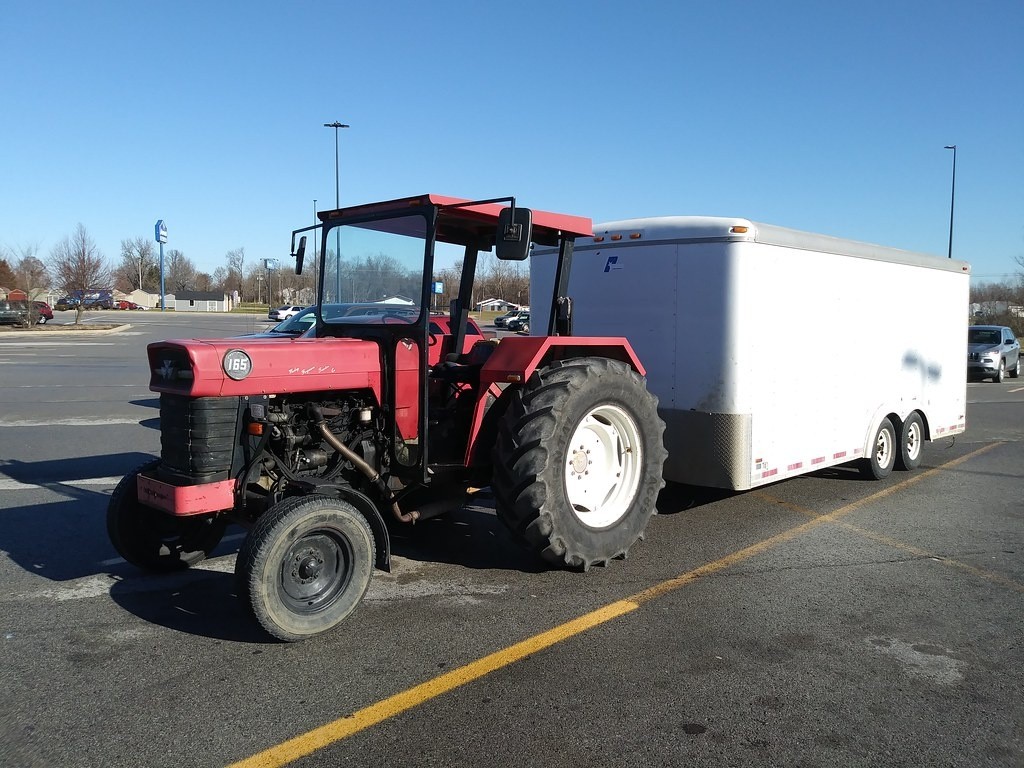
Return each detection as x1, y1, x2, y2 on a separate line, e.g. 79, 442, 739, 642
298, 314, 485, 366
0, 300, 53, 325
967, 325, 1021, 383
494, 310, 529, 328
231, 303, 420, 340
508, 313, 530, 331
268, 305, 306, 322
57, 298, 137, 310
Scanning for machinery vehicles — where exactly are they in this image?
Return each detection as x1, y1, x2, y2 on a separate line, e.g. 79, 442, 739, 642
104, 192, 973, 642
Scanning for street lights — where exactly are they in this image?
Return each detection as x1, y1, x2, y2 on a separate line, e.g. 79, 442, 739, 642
324, 120, 350, 304
944, 145, 956, 258
